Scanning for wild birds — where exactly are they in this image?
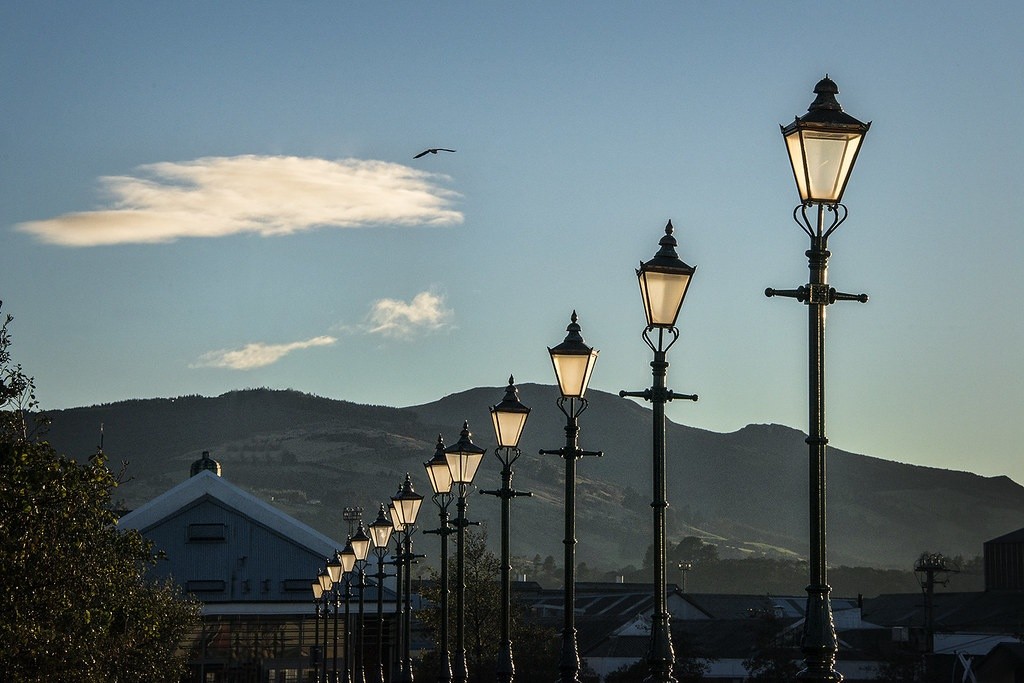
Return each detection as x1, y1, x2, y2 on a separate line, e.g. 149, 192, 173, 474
413, 148, 456, 160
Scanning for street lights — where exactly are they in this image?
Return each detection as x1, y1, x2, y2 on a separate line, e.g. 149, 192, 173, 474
764, 72, 871, 683
538, 309, 605, 683
310, 502, 398, 683
679, 560, 692, 594
422, 419, 488, 683
478, 374, 535, 683
367, 501, 398, 683
620, 219, 699, 683
387, 472, 425, 683
